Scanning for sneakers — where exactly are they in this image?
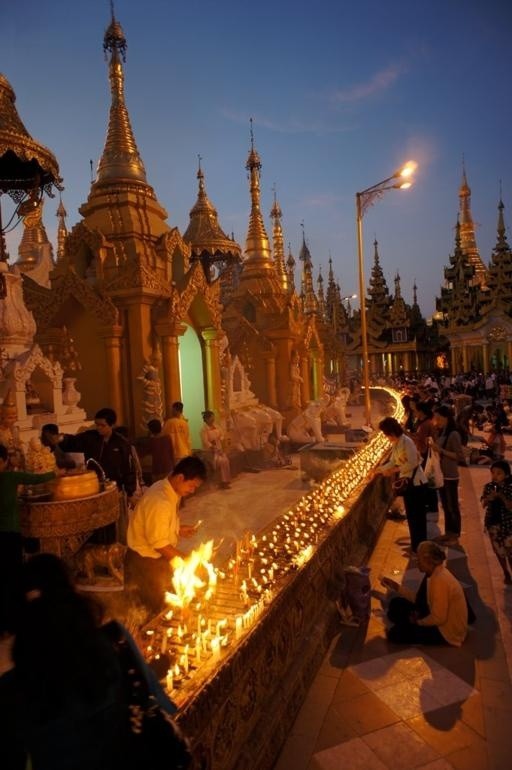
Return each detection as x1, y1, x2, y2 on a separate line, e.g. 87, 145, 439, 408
401, 534, 460, 557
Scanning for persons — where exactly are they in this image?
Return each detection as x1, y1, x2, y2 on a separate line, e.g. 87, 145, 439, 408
37, 400, 228, 548
123, 456, 207, 614
8, 552, 117, 768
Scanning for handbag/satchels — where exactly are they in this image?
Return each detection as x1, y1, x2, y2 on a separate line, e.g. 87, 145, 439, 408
392, 476, 414, 497
126, 679, 194, 769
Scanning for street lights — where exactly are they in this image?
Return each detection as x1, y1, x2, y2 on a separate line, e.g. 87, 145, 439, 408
356, 162, 421, 430
